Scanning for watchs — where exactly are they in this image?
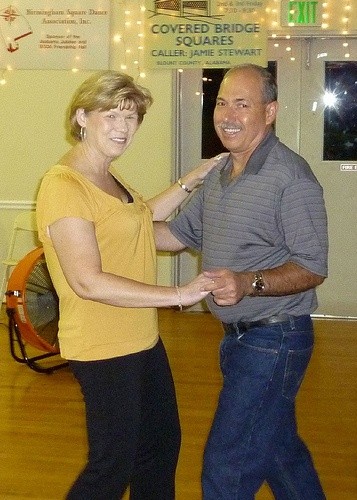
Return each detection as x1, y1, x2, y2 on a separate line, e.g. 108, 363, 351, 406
248, 270, 266, 297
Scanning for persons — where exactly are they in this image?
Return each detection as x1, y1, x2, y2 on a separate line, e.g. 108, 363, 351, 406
152, 63, 328, 500
35, 70, 230, 500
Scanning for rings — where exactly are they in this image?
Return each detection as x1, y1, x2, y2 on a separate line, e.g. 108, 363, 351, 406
211, 280, 215, 284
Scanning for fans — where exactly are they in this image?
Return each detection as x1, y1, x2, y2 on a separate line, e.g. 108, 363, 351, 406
5, 247, 67, 374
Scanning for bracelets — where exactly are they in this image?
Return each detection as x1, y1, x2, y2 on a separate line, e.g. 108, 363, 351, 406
177, 177, 192, 194
176, 285, 183, 312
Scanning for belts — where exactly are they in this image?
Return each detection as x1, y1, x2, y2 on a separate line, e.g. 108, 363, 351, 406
222, 314, 309, 335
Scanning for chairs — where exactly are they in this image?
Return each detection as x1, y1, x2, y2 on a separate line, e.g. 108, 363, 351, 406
0, 212, 38, 312
